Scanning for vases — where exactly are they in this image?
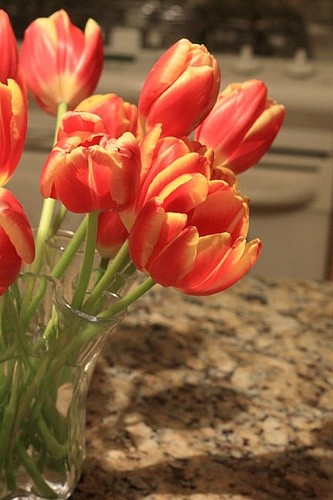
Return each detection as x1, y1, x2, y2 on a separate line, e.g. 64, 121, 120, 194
0, 229, 138, 499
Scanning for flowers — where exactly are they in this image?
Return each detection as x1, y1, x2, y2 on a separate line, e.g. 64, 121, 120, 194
0, 8, 287, 492
